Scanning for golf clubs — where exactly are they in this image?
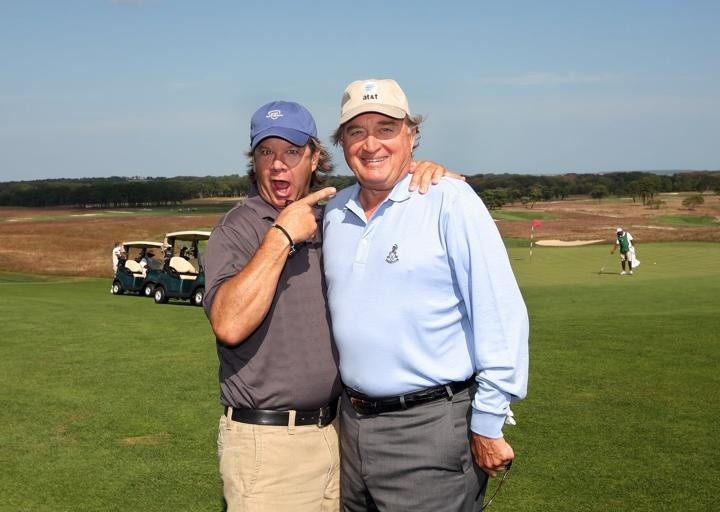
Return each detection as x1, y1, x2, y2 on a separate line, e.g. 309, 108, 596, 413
599, 254, 613, 273
179, 243, 196, 258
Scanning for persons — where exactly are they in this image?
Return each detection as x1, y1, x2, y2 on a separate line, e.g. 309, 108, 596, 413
203, 101, 468, 511
322, 77, 529, 512
110, 241, 126, 294
609, 227, 635, 274
138, 249, 156, 276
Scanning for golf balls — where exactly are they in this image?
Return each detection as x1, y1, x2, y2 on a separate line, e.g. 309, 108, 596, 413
653, 261, 656, 264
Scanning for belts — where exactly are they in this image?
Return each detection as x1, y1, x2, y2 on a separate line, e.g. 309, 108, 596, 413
342, 373, 476, 415
224, 405, 339, 428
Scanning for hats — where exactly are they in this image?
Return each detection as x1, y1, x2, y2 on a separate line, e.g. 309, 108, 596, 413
341, 79, 410, 124
251, 102, 317, 149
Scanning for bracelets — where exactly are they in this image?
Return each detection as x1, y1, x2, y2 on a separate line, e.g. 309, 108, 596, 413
270, 222, 297, 257
